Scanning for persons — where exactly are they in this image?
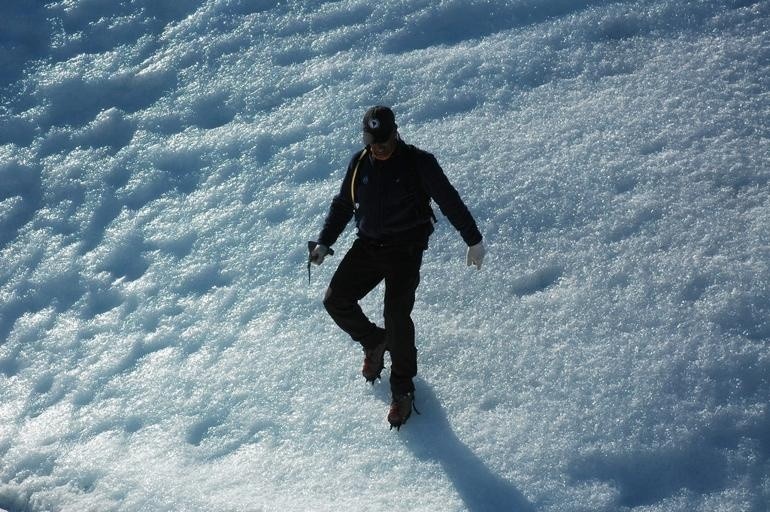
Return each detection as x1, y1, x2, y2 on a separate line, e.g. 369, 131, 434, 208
307, 103, 489, 431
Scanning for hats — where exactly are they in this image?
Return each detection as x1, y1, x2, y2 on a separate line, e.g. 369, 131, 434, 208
362, 106, 398, 145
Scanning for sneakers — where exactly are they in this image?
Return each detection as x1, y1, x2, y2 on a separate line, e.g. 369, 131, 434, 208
362, 326, 389, 383
387, 391, 415, 426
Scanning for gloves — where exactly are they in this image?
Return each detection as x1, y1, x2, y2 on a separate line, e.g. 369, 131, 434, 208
465, 239, 485, 271
308, 243, 329, 266
